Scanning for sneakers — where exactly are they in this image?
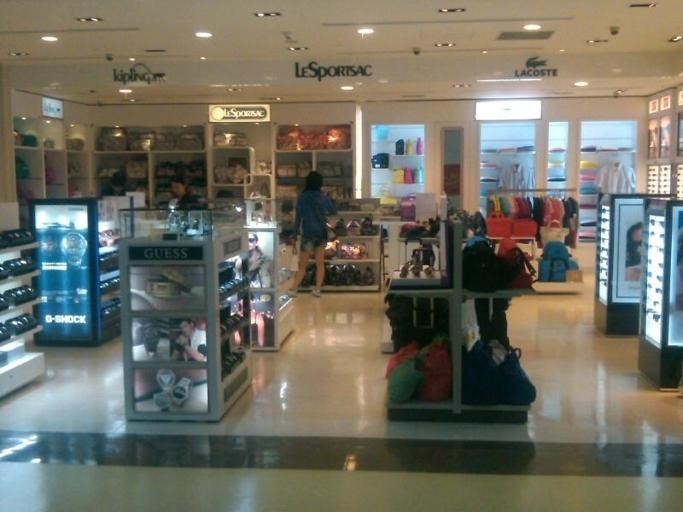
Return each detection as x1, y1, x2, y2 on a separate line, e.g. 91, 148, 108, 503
287, 290, 297, 298
312, 289, 322, 297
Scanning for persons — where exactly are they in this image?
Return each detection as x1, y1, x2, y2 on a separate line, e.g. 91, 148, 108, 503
288, 170, 336, 298
101, 172, 128, 195
248, 233, 272, 290
133, 180, 148, 205
168, 177, 203, 209
168, 319, 205, 363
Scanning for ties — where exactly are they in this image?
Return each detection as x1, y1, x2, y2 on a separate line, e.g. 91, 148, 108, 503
188, 336, 192, 346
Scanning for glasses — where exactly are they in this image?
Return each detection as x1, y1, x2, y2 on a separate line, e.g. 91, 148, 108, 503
647, 168, 683, 200
249, 238, 257, 243
642, 218, 666, 323
596, 207, 611, 287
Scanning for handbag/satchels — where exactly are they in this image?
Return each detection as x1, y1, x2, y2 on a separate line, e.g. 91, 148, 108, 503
486, 210, 570, 246
462, 334, 537, 406
396, 137, 424, 155
393, 169, 423, 184
385, 335, 450, 402
304, 219, 381, 286
462, 237, 534, 290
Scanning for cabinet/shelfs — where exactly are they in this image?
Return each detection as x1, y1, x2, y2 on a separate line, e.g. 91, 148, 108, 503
0, 88, 299, 422
593, 87, 682, 392
383, 222, 537, 424
300, 87, 592, 294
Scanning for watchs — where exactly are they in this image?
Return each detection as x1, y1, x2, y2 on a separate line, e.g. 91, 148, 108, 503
39, 229, 89, 268
151, 369, 190, 412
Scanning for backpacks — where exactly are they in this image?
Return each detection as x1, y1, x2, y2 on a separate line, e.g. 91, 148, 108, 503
541, 241, 571, 282
